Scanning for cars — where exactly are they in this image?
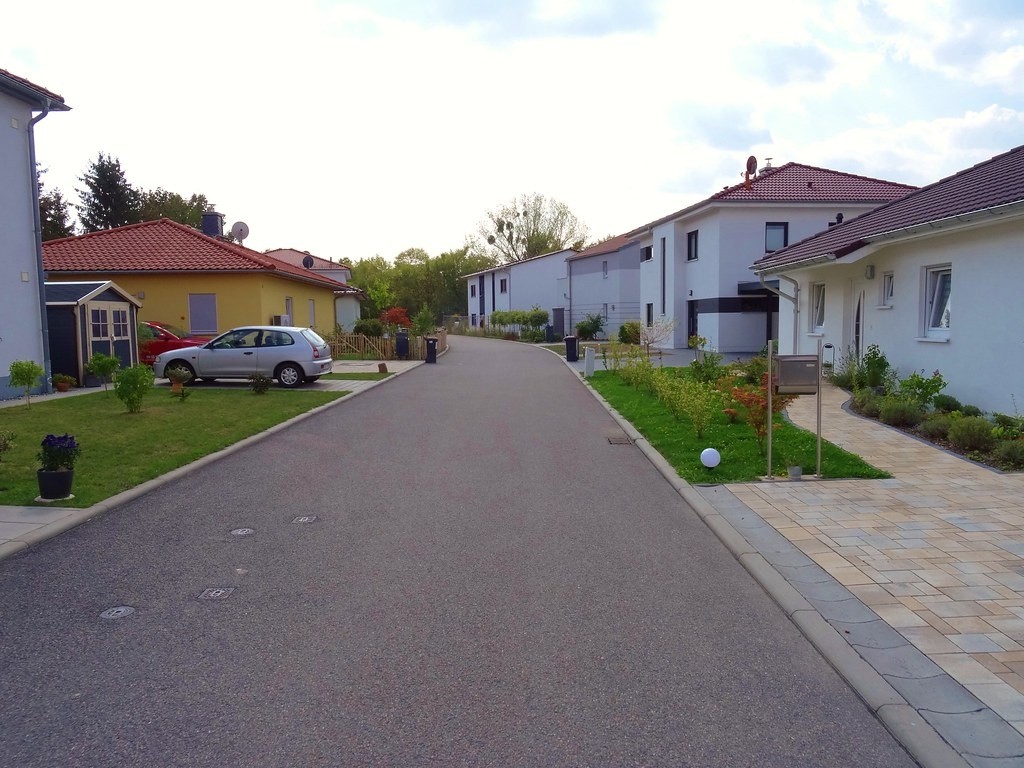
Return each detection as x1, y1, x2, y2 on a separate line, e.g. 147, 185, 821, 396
137, 322, 220, 366
154, 325, 333, 387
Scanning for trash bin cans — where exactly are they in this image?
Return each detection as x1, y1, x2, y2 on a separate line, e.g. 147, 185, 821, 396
545, 325, 553, 341
423, 335, 439, 364
395, 332, 409, 360
563, 336, 579, 362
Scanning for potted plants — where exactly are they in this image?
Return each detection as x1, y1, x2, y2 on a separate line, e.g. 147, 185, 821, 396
48, 372, 77, 393
166, 367, 192, 394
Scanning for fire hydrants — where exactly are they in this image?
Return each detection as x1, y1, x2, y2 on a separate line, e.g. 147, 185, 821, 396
582, 347, 586, 357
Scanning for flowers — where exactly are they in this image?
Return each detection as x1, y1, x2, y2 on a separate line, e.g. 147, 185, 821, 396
36, 431, 82, 469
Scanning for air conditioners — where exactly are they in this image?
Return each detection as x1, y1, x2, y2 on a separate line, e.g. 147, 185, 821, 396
273, 315, 290, 340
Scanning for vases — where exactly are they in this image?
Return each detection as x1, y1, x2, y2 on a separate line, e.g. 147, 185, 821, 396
37, 470, 74, 498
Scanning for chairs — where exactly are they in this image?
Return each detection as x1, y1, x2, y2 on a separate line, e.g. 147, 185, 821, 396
262, 336, 273, 345
